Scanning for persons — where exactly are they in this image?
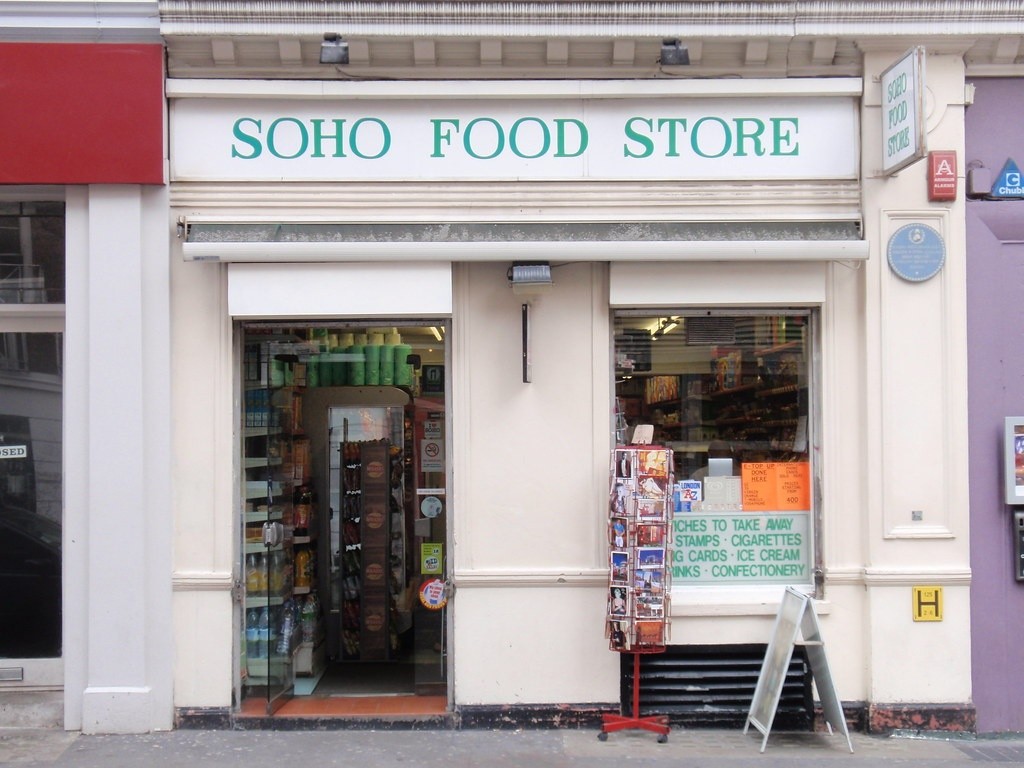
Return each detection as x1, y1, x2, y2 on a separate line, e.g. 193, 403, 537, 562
690, 440, 742, 501
611, 622, 626, 650
612, 589, 626, 615
616, 451, 631, 478
611, 483, 627, 516
639, 477, 662, 495
614, 519, 625, 547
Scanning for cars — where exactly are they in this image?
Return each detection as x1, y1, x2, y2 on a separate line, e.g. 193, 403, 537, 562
0, 507, 62, 659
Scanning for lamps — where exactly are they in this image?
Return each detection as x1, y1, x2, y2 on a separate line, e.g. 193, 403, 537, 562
507, 261, 556, 309
660, 38, 690, 65
320, 32, 349, 64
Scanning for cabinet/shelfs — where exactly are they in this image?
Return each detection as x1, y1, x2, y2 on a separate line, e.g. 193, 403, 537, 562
339, 439, 403, 664
243, 334, 316, 695
710, 339, 801, 442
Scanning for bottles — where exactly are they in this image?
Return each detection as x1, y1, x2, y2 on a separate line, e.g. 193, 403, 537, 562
259, 608, 274, 658
248, 553, 293, 595
295, 488, 310, 536
276, 608, 294, 657
302, 596, 320, 642
296, 548, 314, 586
246, 608, 259, 658
